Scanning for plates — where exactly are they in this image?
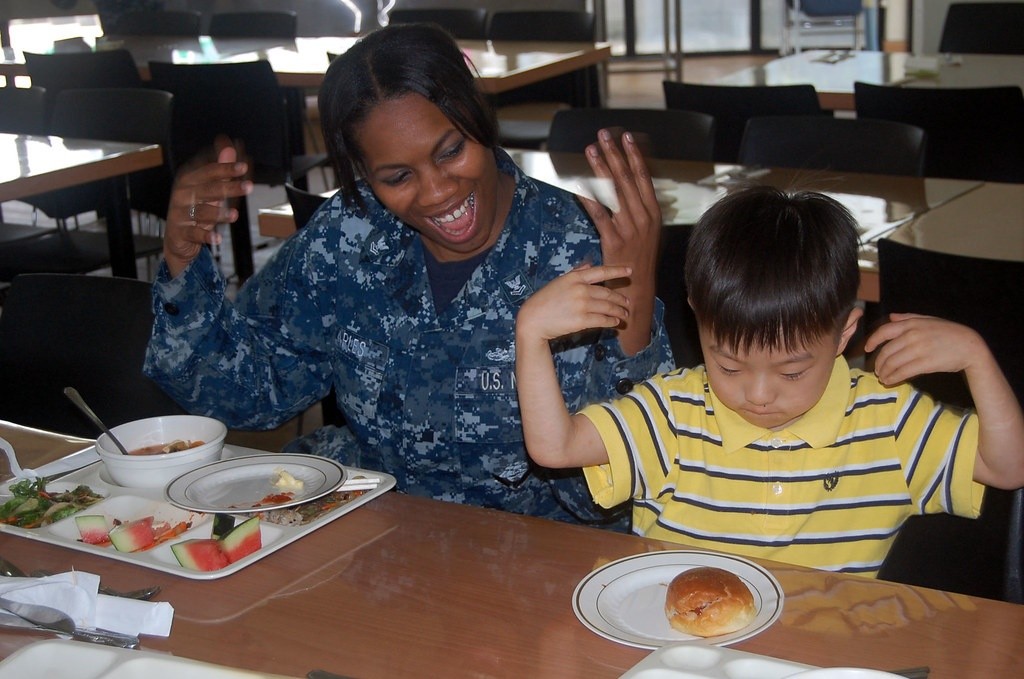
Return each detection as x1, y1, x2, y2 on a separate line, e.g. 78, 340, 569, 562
164, 453, 348, 513
571, 550, 784, 651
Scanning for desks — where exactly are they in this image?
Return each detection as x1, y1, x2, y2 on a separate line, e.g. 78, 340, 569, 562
0, 133, 163, 279
703, 48, 1024, 110
0, 422, 1023, 678
258, 147, 1024, 303
0, 24, 610, 194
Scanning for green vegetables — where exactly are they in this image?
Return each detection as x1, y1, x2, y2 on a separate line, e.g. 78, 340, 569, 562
0, 476, 100, 529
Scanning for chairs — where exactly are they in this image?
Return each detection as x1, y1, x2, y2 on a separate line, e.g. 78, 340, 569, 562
1, 0, 1022, 609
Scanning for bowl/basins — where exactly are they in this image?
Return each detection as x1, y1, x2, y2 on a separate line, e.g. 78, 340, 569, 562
94, 414, 227, 489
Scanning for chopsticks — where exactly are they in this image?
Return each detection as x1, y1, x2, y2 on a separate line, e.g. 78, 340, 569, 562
890, 666, 931, 679
335, 478, 382, 492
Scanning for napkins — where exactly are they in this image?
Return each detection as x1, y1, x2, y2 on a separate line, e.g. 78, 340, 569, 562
1, 571, 174, 638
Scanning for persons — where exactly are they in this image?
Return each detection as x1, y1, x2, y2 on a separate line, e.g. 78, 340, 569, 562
143, 21, 677, 533
515, 165, 1024, 579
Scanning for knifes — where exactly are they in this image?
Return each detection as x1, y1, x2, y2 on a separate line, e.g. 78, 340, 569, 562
0, 598, 76, 634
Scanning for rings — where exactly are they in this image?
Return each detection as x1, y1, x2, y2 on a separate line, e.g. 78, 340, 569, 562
190, 203, 200, 222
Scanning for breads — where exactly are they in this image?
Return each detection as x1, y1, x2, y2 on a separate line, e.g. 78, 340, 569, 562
664, 566, 756, 638
